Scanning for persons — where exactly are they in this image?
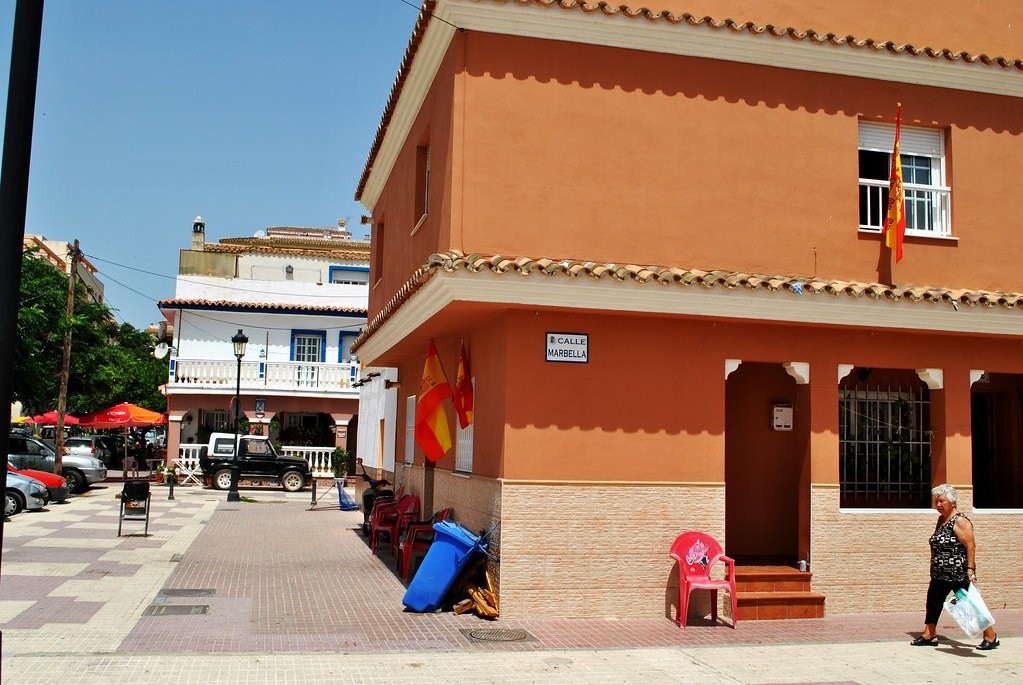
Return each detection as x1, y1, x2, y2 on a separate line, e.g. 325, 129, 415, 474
909, 483, 1002, 650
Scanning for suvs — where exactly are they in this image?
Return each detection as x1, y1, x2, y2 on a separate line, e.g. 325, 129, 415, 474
8, 431, 108, 492
40, 426, 73, 447
199, 431, 311, 490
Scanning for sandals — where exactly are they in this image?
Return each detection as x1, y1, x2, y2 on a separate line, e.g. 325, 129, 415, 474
976, 633, 1000, 650
910, 636, 938, 646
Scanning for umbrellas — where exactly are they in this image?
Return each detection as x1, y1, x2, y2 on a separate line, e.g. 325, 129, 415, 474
78, 402, 169, 478
21, 410, 78, 427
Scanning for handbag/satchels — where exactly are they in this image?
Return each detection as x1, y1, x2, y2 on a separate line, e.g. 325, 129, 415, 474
942, 574, 995, 639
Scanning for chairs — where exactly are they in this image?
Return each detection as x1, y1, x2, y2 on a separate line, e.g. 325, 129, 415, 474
670, 532, 737, 629
396, 511, 442, 575
118, 480, 152, 537
398, 506, 452, 583
121, 459, 139, 479
369, 486, 420, 559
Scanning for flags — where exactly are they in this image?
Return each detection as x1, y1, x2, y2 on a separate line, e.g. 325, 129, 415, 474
415, 339, 454, 462
882, 102, 905, 264
452, 338, 473, 433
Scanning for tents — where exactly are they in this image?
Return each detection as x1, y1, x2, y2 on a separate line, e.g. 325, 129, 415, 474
11, 416, 32, 424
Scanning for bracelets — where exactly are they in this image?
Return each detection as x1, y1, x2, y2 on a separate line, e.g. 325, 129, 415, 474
966, 566, 975, 571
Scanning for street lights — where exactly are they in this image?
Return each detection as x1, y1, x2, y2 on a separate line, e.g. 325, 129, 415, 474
228, 329, 249, 502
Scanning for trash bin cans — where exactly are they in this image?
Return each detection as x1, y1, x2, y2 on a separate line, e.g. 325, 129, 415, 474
123, 481, 150, 515
401, 520, 489, 613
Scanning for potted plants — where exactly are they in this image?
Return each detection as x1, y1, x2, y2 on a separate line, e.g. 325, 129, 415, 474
155, 465, 164, 483
332, 446, 351, 487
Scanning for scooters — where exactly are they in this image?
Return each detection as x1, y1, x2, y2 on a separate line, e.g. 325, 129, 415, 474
351, 457, 398, 539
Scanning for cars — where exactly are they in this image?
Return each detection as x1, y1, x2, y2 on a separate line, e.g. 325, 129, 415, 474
92, 428, 166, 458
7, 460, 70, 503
4, 471, 50, 515
60, 435, 110, 464
